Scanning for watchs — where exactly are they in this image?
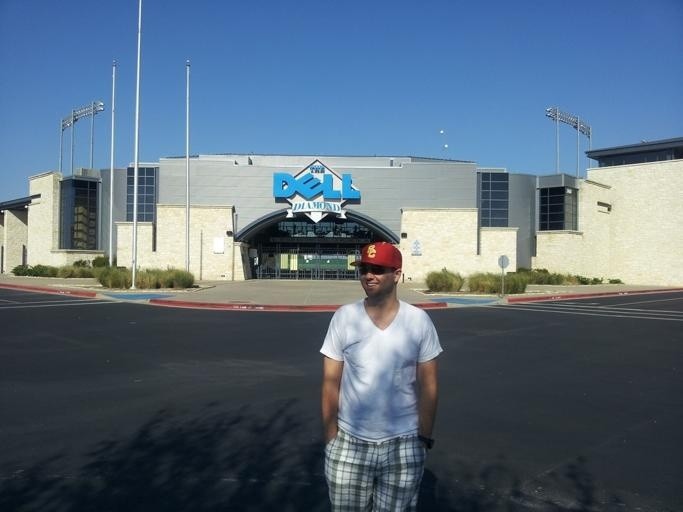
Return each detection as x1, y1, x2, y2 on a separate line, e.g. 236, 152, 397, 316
418, 434, 435, 449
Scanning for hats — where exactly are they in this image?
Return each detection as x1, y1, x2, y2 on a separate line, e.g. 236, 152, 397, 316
349, 240, 403, 270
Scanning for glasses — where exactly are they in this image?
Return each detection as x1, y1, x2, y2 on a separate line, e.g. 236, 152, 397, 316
359, 267, 386, 275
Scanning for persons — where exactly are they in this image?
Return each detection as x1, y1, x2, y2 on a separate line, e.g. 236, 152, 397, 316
319, 242, 445, 511
